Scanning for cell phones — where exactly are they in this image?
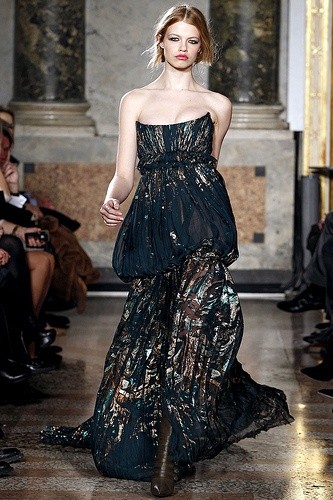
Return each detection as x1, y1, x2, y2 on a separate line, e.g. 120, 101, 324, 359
24, 230, 50, 243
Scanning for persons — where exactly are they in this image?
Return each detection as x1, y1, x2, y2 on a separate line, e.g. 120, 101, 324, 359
275, 201, 332, 407
48, 1, 291, 491
0, 104, 91, 473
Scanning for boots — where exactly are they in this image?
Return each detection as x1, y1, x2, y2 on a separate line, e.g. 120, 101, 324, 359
150, 411, 180, 498
179, 460, 197, 477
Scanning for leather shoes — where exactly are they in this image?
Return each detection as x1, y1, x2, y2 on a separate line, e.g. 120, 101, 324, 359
6, 353, 56, 376
0, 362, 33, 386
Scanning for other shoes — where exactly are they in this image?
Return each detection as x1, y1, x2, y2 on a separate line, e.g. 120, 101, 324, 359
44, 346, 63, 361
40, 329, 56, 345
317, 388, 333, 400
0, 460, 14, 477
302, 321, 333, 346
276, 286, 325, 313
302, 350, 333, 381
1, 446, 22, 464
